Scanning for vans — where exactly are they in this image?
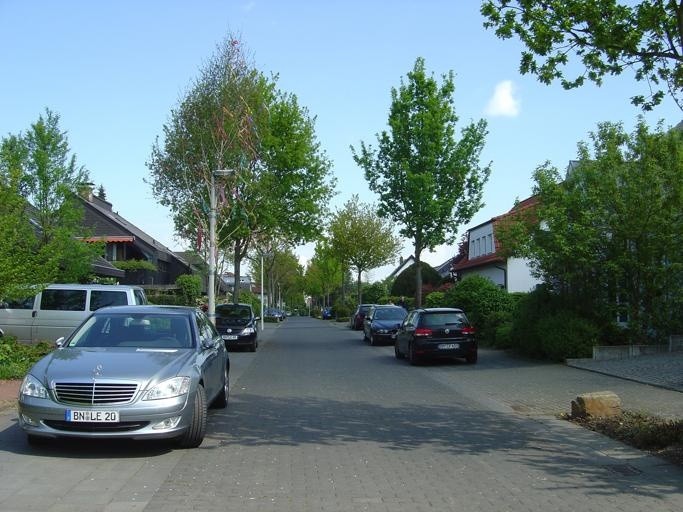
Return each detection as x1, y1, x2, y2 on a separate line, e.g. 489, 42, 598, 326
0, 283, 149, 347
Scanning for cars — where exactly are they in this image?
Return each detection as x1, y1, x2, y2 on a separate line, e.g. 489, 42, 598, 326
322, 307, 335, 319
394, 308, 478, 366
264, 310, 283, 323
351, 304, 409, 345
204, 303, 260, 352
18, 305, 230, 448
